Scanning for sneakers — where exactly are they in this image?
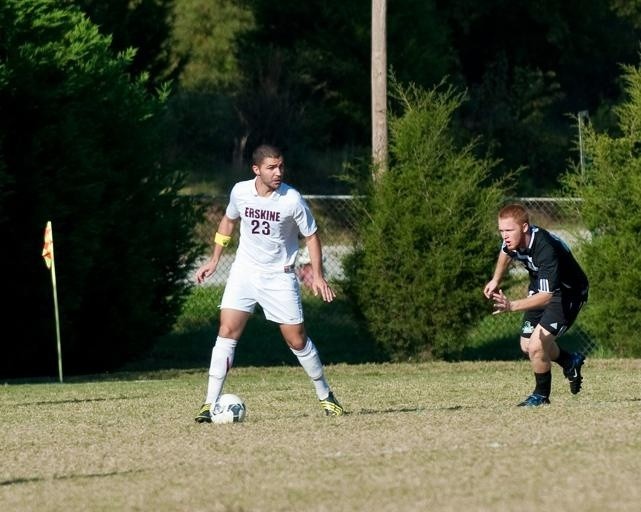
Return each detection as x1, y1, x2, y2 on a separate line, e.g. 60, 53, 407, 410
195, 403, 211, 423
564, 352, 584, 394
320, 391, 344, 417
517, 394, 550, 406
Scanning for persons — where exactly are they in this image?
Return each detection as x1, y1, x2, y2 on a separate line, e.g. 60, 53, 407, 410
483, 203, 589, 408
192, 144, 345, 423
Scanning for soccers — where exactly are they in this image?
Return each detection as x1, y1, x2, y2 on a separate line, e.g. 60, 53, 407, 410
209, 394, 246, 425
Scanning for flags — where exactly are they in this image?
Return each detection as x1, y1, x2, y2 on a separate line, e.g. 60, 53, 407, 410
42, 221, 53, 272
